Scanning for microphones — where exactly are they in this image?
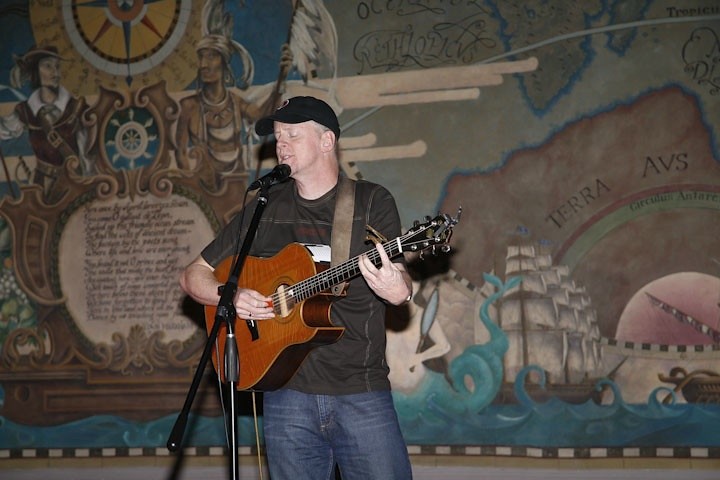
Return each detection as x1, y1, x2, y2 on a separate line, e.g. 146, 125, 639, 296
247, 164, 291, 191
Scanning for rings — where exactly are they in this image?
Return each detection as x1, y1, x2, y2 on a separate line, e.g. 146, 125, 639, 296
248, 311, 253, 317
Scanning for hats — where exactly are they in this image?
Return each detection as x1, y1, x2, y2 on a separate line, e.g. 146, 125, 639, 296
255, 96, 340, 139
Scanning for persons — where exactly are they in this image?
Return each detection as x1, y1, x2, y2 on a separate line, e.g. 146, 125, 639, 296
179, 97, 413, 480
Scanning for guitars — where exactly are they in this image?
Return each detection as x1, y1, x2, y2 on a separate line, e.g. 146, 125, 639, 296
204, 212, 455, 393
